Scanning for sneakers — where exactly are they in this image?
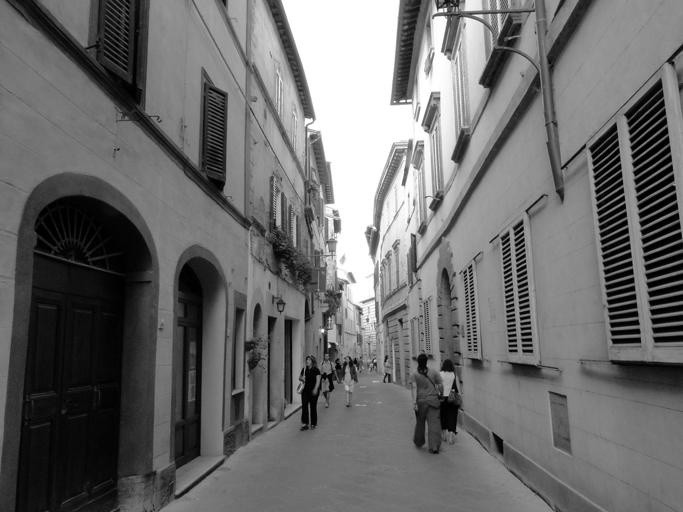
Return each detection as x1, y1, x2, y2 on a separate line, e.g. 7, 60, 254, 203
300, 425, 315, 431
325, 402, 329, 408
345, 402, 352, 407
413, 429, 456, 455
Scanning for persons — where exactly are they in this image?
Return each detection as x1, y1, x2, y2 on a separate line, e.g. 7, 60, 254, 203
296, 353, 321, 431
334, 355, 377, 384
438, 357, 462, 443
317, 352, 339, 408
338, 355, 358, 407
381, 354, 391, 383
410, 352, 443, 455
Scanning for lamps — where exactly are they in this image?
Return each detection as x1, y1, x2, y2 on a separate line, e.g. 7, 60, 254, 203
432, 1, 464, 18
272, 294, 286, 314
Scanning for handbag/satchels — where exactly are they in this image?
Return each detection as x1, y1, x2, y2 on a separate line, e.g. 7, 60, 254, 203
322, 378, 330, 392
296, 375, 305, 394
447, 389, 463, 408
